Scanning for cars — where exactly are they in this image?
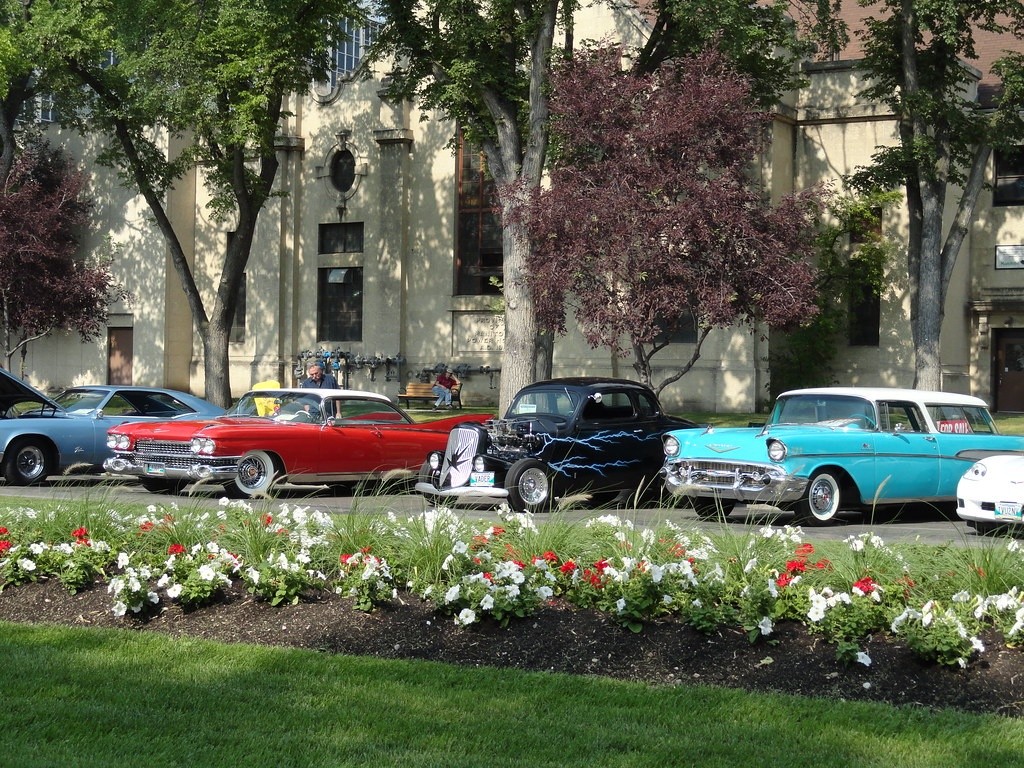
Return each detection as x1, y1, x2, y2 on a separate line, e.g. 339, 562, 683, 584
658, 387, 1023, 525
956, 456, 1024, 532
0, 368, 226, 485
415, 377, 698, 512
102, 389, 494, 498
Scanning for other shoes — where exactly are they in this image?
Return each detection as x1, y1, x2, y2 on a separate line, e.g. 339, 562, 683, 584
432, 404, 437, 411
448, 405, 456, 410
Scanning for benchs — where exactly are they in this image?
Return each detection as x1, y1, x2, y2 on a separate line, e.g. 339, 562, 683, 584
396, 383, 464, 409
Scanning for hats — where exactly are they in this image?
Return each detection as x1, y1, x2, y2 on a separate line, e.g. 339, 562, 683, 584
447, 369, 453, 374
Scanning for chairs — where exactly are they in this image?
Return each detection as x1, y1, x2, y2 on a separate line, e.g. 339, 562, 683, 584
583, 398, 608, 420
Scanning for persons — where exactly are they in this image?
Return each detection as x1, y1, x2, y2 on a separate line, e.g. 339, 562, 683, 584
301, 362, 343, 419
431, 369, 461, 411
